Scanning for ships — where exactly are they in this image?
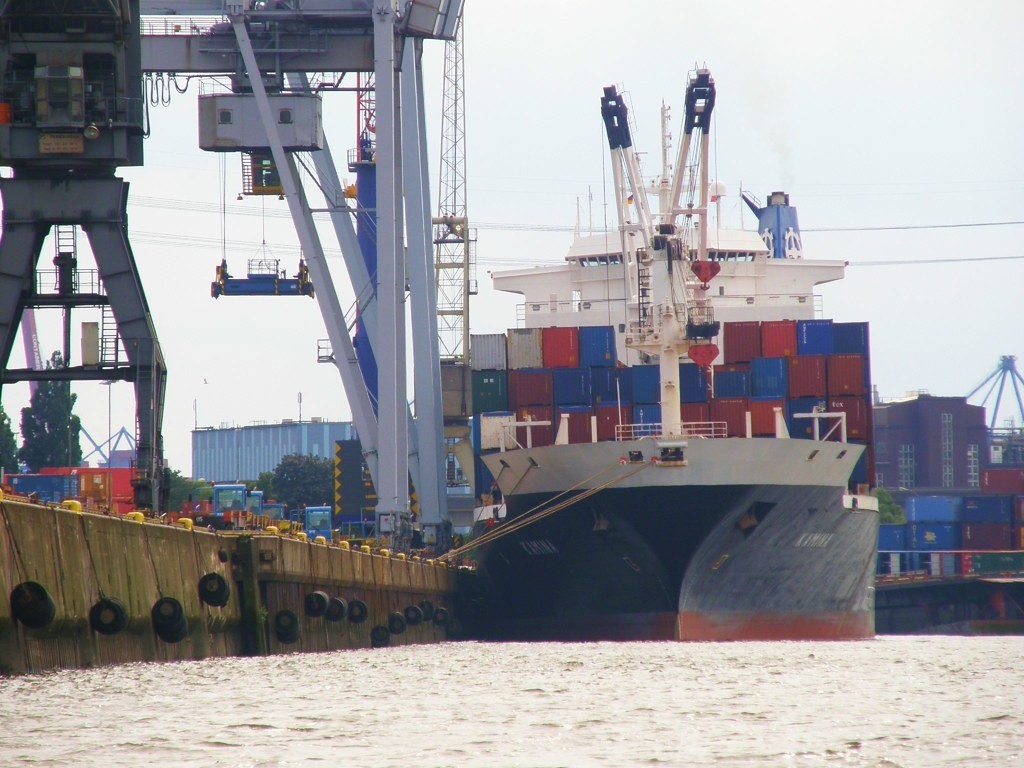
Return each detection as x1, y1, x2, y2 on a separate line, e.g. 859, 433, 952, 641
458, 58, 883, 641
